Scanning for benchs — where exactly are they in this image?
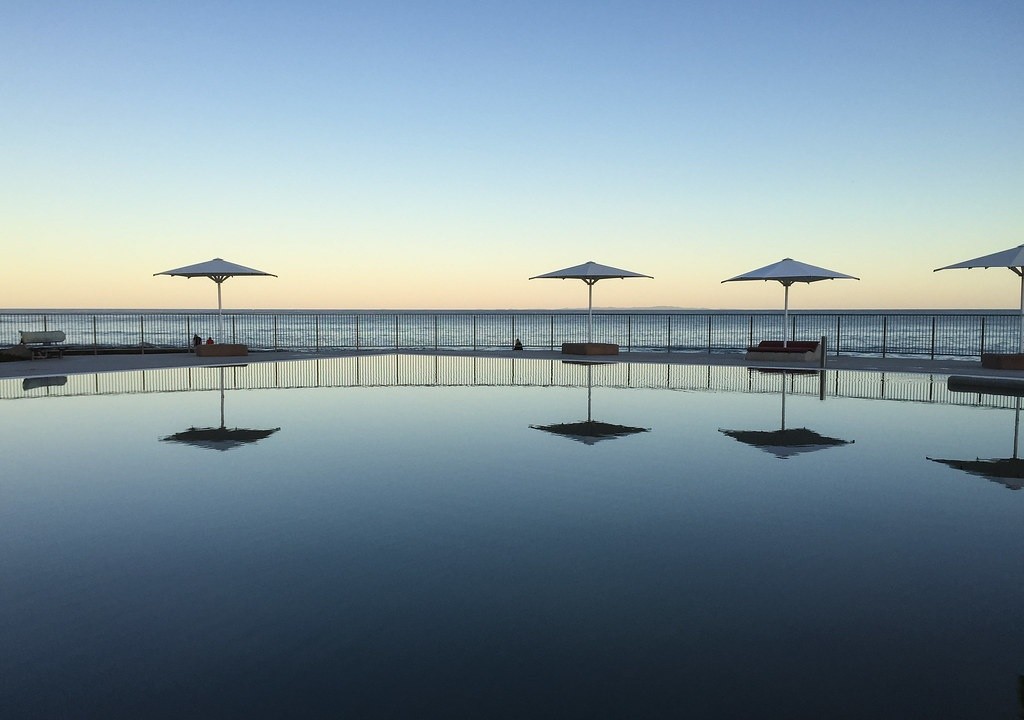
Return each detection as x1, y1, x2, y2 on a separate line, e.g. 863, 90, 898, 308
19, 330, 75, 360
745, 340, 823, 363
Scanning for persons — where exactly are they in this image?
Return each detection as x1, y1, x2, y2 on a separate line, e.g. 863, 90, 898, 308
193, 334, 202, 347
207, 337, 214, 345
513, 339, 524, 351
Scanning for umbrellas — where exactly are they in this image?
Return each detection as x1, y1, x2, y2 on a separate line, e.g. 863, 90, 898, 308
527, 364, 652, 446
152, 258, 278, 344
158, 368, 283, 452
717, 370, 856, 460
927, 395, 1024, 491
721, 258, 861, 348
528, 261, 654, 343
933, 244, 1023, 354
